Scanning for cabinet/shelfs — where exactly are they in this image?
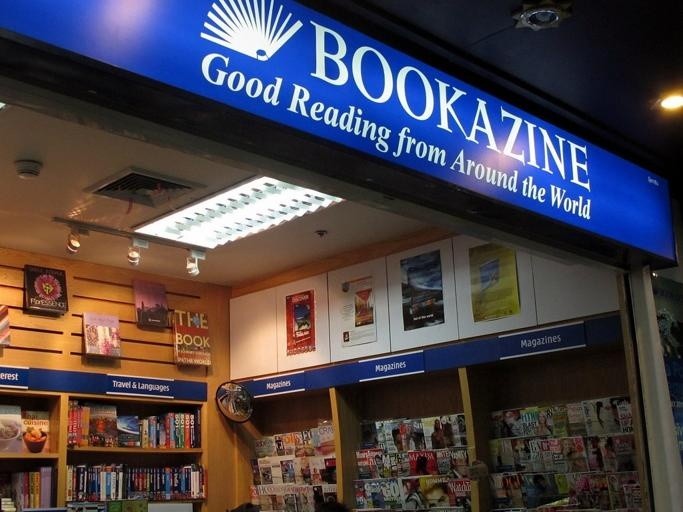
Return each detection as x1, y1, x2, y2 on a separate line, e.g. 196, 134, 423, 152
0, 386, 208, 512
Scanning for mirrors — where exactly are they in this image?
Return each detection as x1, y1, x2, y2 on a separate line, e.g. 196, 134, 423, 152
216, 383, 254, 423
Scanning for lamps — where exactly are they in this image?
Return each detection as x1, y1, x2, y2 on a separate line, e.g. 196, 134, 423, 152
47, 216, 216, 277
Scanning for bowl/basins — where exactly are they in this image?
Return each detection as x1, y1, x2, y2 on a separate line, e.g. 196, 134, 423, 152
0, 424, 20, 451
22, 430, 48, 454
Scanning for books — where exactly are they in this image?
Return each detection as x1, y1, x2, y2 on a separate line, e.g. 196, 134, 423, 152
172, 309, 212, 367
0, 304, 10, 347
82, 311, 121, 359
132, 278, 169, 328
23, 264, 68, 312
